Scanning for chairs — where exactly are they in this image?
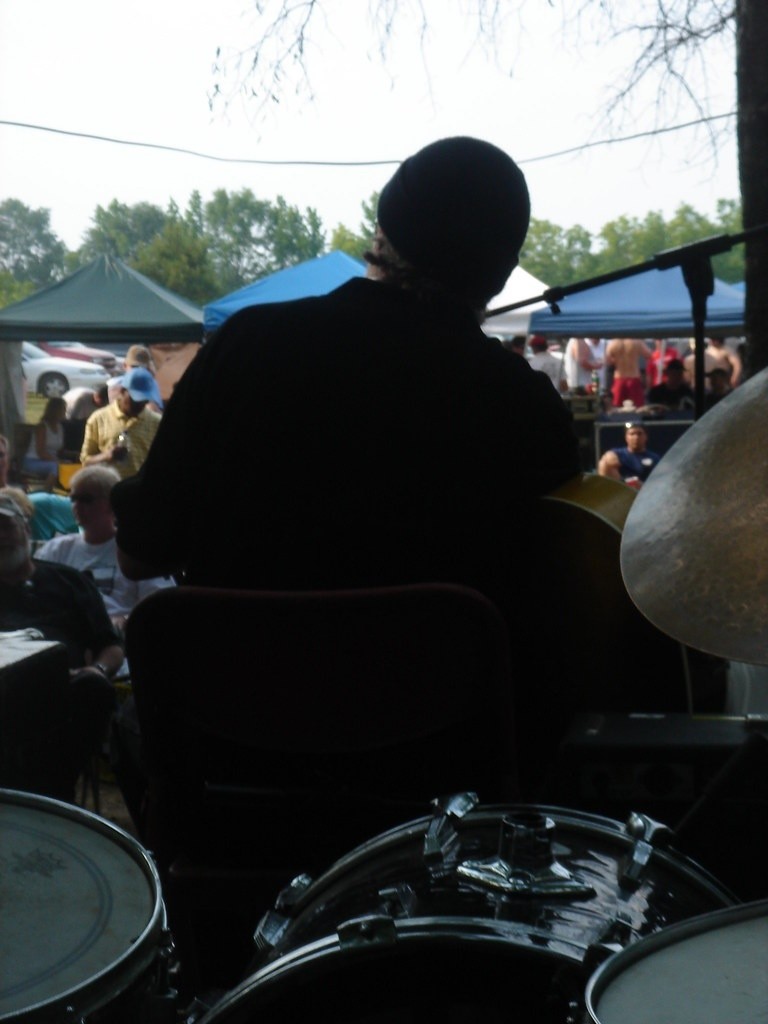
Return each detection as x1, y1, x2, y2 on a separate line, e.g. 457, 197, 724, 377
126, 582, 520, 985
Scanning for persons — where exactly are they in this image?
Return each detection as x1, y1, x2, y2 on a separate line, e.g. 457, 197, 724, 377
115, 137, 587, 780
0, 335, 747, 800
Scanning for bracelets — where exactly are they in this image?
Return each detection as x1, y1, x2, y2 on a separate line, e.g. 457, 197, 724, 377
95, 662, 108, 673
122, 613, 129, 619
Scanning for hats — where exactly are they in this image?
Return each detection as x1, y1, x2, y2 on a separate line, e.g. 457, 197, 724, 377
0, 496, 25, 518
126, 344, 151, 365
377, 137, 530, 298
120, 367, 164, 408
667, 359, 686, 370
705, 368, 727, 377
528, 336, 545, 345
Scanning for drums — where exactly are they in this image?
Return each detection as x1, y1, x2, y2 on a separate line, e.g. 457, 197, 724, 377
584, 884, 768, 1024
0, 788, 178, 1024
179, 794, 740, 1024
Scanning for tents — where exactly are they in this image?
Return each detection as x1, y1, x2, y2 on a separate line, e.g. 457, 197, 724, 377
0, 247, 749, 422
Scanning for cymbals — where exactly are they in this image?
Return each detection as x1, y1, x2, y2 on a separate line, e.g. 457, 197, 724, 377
618, 364, 767, 669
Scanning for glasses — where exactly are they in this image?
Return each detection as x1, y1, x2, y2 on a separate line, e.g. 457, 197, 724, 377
70, 492, 105, 504
